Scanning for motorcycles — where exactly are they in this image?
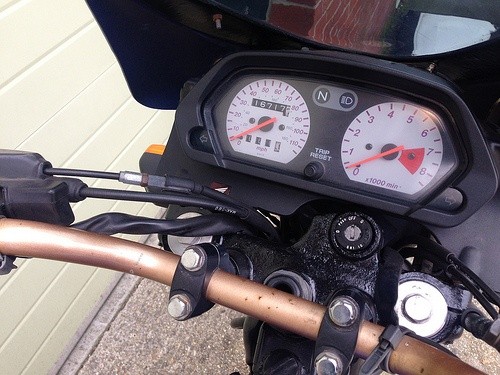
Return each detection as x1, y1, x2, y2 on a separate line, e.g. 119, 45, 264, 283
0, 46, 500, 375
